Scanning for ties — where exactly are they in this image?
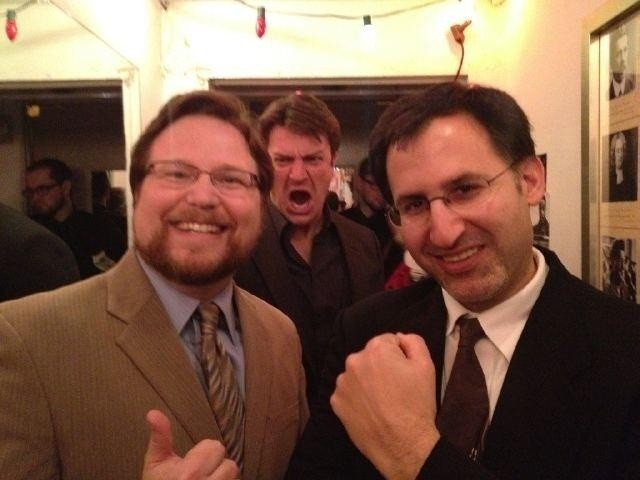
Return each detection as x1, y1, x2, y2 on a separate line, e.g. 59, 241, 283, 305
436, 316, 489, 458
193, 302, 244, 473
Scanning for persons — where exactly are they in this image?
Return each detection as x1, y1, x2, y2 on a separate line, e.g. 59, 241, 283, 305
606, 25, 638, 103
91, 169, 128, 249
21, 159, 126, 281
325, 191, 346, 213
3, 204, 80, 302
607, 131, 637, 203
1, 86, 312, 480
599, 236, 632, 303
337, 158, 429, 291
276, 78, 639, 479
229, 92, 386, 413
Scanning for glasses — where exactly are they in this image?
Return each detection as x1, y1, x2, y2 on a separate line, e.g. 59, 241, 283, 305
144, 161, 261, 195
389, 159, 519, 228
21, 183, 59, 196
